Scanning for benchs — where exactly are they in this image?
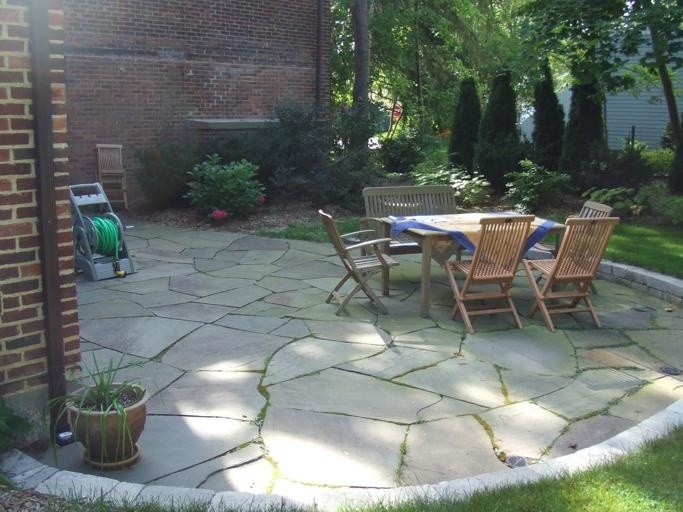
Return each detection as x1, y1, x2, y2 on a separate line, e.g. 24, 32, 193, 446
360, 186, 467, 298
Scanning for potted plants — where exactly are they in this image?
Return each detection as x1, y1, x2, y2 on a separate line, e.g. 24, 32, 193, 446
40, 341, 165, 477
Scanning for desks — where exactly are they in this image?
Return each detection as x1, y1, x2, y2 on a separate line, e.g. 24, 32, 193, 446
383, 211, 567, 319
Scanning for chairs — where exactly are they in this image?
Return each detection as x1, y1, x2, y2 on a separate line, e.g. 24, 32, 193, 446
522, 216, 619, 332
442, 214, 535, 334
95, 144, 129, 212
534, 200, 613, 295
319, 209, 398, 315
